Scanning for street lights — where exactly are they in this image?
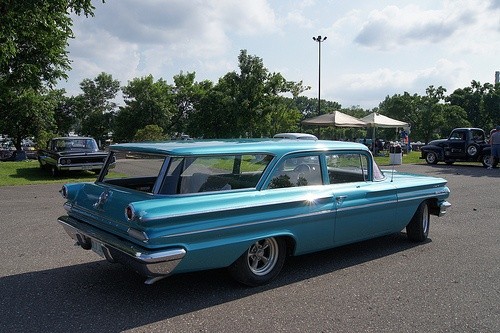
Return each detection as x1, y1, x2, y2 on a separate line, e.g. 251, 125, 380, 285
312, 35, 328, 141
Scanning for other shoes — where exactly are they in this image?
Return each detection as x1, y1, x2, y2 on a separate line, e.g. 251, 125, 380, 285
487, 167, 493, 170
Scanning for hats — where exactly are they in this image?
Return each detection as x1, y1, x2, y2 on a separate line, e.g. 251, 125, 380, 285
496, 126, 500, 130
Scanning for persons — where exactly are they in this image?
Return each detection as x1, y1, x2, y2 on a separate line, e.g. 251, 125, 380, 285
486, 126, 500, 170
336, 135, 408, 156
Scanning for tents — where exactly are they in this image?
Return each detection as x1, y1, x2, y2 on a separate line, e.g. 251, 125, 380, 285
302, 111, 409, 156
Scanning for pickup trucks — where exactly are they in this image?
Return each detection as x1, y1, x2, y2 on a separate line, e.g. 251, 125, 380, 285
418, 127, 500, 168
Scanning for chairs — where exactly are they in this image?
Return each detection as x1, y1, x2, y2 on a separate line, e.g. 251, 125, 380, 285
184, 172, 232, 191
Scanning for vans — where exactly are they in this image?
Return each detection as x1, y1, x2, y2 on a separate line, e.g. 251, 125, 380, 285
273, 133, 320, 143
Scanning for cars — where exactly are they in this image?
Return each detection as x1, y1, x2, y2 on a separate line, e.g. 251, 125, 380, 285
55, 137, 452, 291
36, 135, 117, 177
